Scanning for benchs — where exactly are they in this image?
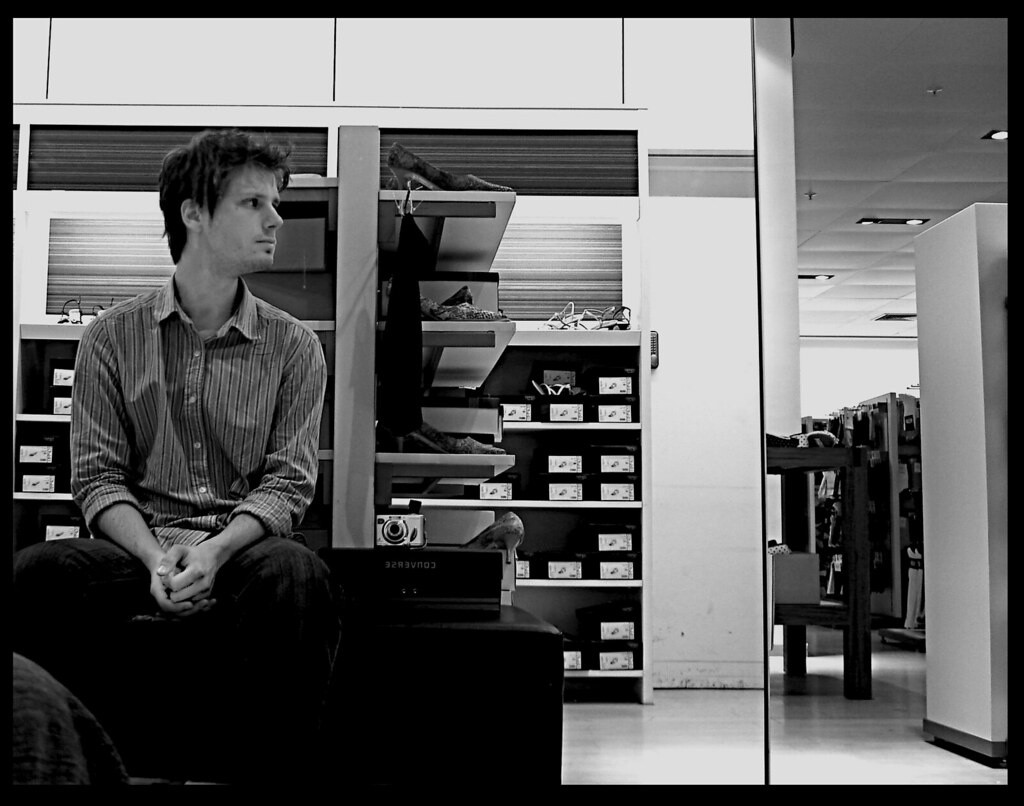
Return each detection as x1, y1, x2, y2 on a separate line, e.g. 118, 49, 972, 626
77, 605, 565, 780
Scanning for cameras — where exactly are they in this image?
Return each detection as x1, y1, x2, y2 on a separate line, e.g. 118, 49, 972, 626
376, 513, 425, 547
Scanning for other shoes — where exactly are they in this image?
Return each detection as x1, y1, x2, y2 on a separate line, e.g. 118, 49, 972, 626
398, 419, 506, 454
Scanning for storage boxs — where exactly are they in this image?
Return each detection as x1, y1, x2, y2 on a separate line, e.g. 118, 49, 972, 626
771, 551, 821, 602
14, 354, 83, 542
315, 545, 505, 620
475, 360, 636, 670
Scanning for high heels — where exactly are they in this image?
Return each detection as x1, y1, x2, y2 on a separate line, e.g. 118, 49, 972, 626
458, 511, 525, 564
388, 142, 513, 191
386, 283, 508, 322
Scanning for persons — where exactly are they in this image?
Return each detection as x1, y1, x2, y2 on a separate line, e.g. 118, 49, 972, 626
8, 131, 342, 790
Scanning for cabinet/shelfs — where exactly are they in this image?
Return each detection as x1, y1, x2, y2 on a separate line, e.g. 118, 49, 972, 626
763, 388, 927, 696
491, 326, 655, 702
15, 323, 80, 503
254, 123, 519, 604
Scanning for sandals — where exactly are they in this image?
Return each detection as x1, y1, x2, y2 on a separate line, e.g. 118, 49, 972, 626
532, 378, 573, 396
536, 302, 582, 329
580, 304, 631, 329
